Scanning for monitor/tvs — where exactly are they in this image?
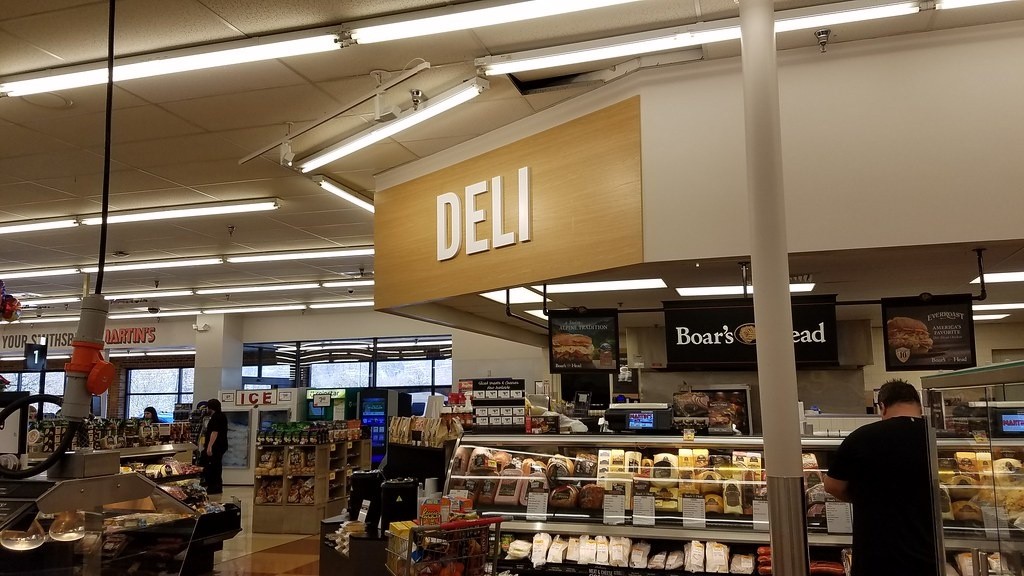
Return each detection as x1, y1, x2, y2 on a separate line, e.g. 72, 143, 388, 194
626, 411, 658, 430
361, 397, 385, 447
308, 402, 324, 415
992, 409, 1024, 436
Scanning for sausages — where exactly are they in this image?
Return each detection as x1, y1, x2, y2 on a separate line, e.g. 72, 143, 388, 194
757, 547, 845, 576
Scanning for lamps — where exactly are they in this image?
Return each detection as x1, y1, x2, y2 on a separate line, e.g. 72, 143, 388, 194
1, 0, 1024, 327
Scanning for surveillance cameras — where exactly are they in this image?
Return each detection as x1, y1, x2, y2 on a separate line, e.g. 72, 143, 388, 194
347, 288, 354, 294
148, 308, 160, 314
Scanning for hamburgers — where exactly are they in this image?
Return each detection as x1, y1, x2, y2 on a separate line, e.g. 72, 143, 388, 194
553, 332, 595, 362
888, 317, 933, 355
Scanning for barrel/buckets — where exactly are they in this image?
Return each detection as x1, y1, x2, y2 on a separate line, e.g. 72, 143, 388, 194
381, 477, 418, 542
350, 470, 384, 526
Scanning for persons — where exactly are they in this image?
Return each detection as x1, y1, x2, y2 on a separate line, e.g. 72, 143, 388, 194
823, 379, 938, 576
203, 399, 229, 495
143, 407, 160, 423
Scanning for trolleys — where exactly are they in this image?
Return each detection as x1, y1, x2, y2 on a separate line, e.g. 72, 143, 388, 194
384, 515, 514, 576
